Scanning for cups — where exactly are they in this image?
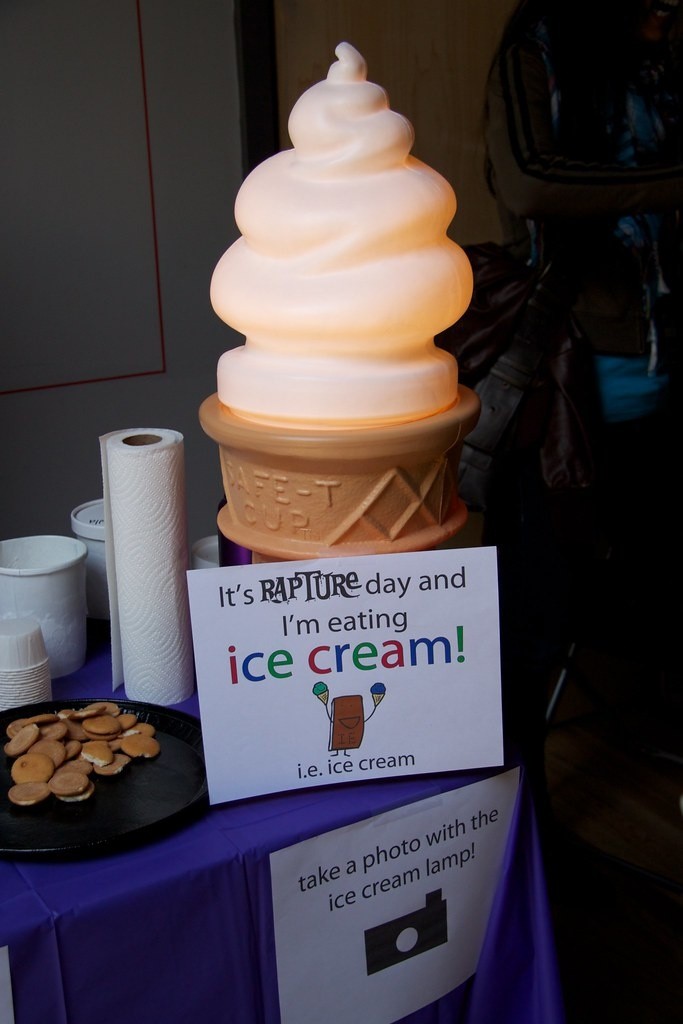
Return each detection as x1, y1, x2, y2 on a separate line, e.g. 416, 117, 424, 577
71, 499, 112, 619
1, 536, 88, 680
0, 620, 54, 712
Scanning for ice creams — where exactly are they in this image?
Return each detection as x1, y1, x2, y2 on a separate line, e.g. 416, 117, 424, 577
198, 43, 479, 565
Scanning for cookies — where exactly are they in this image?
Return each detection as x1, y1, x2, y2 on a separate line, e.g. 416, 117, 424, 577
5, 704, 159, 804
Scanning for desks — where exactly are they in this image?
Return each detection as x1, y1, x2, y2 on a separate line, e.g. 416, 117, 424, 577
0, 616, 570, 1024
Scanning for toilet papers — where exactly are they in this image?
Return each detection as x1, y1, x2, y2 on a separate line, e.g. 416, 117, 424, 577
98, 426, 196, 707
